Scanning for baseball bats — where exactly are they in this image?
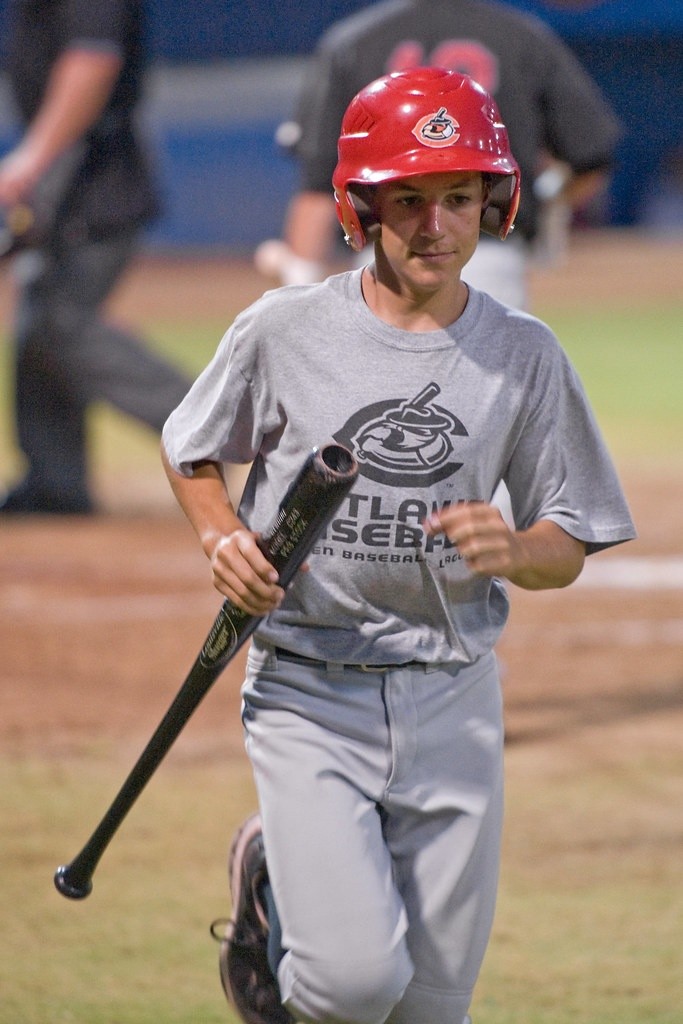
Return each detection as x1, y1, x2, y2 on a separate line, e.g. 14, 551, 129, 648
51, 442, 359, 902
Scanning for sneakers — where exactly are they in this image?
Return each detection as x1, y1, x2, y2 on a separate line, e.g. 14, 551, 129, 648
1, 494, 91, 513
210, 812, 298, 1024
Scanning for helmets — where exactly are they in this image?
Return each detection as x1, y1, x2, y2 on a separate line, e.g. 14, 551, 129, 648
332, 66, 522, 252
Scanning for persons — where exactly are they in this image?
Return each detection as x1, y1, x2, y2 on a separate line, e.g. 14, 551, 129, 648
159, 64, 639, 1023
251, 1, 629, 532
0, 0, 193, 513
